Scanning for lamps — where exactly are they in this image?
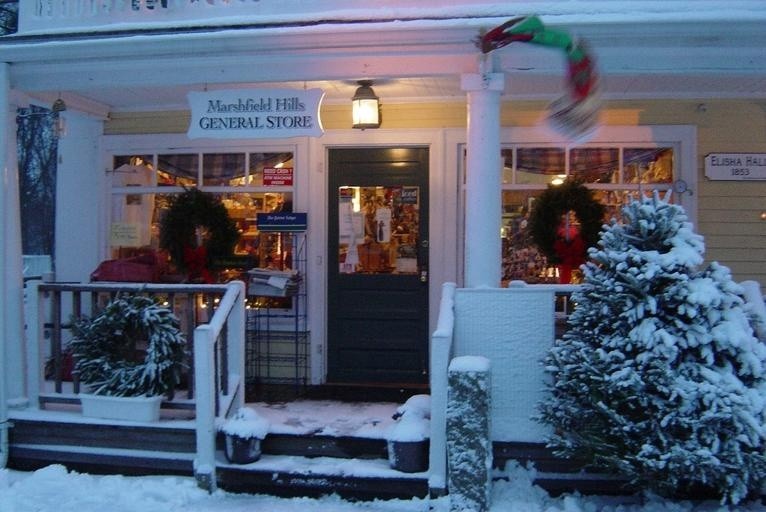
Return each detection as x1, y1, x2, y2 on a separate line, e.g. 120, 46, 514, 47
349, 81, 381, 132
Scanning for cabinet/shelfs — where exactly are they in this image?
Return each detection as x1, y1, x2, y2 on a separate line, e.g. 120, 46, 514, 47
244, 231, 311, 400
